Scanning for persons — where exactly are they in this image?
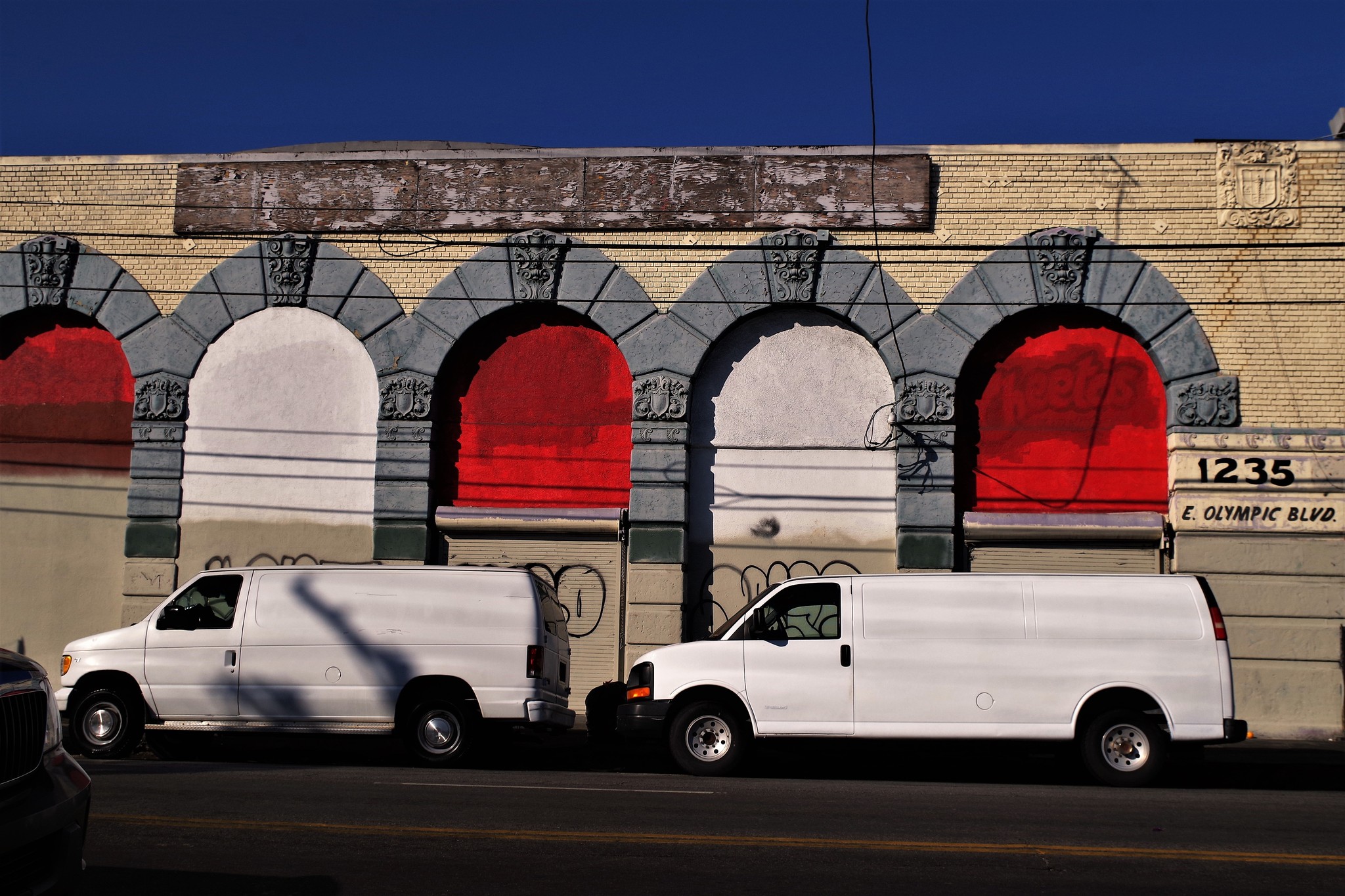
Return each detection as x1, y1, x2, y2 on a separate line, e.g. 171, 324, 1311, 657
198, 584, 239, 628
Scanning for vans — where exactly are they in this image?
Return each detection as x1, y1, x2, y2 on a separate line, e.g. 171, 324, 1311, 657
55, 566, 577, 768
624, 571, 1250, 788
